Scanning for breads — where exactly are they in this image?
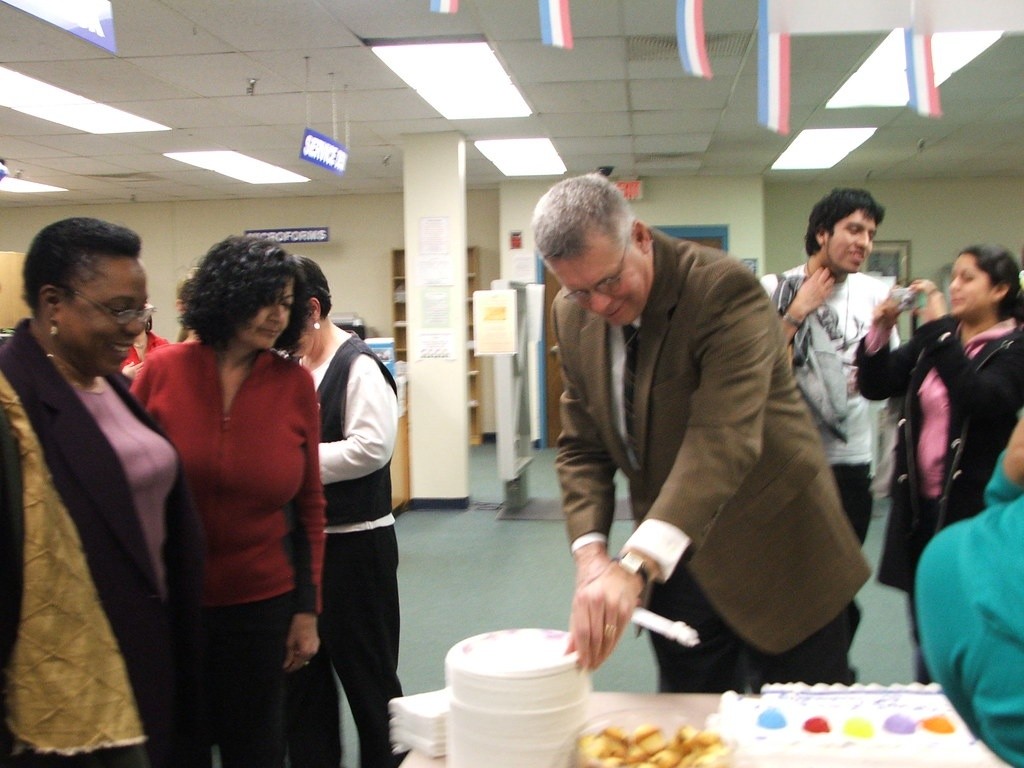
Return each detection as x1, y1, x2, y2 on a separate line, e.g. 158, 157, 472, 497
576, 722, 730, 768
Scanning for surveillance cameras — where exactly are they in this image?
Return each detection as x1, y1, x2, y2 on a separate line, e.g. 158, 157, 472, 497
599, 165, 614, 177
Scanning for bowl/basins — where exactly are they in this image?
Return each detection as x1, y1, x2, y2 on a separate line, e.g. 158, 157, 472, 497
552, 708, 729, 768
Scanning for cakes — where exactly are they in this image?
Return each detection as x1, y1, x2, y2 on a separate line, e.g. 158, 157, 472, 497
714, 681, 989, 768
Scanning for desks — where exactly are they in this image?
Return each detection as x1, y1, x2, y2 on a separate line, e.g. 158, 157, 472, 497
399, 693, 1015, 768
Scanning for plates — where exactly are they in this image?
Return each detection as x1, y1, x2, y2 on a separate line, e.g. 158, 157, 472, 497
445, 629, 593, 768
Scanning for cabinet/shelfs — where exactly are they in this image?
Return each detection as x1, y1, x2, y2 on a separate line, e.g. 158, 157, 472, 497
390, 246, 483, 445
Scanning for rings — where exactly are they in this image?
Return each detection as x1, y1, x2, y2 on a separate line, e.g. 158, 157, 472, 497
303, 660, 310, 666
603, 625, 619, 637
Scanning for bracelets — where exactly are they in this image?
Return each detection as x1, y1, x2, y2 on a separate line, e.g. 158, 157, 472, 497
783, 311, 803, 329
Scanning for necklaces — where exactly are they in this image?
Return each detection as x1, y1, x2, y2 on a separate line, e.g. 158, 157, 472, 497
808, 260, 850, 352
132, 342, 146, 349
55, 365, 104, 394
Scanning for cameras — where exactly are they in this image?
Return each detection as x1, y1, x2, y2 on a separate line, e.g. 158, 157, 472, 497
890, 288, 919, 312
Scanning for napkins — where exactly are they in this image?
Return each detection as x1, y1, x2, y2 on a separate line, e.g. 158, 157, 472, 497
388, 689, 447, 758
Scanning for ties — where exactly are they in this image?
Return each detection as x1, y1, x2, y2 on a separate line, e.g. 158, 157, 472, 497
623, 322, 641, 438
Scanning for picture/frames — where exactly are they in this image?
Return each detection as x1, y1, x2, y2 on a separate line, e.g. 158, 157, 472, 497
862, 240, 910, 289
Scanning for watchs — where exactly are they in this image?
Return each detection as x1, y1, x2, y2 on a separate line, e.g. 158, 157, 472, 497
610, 552, 650, 587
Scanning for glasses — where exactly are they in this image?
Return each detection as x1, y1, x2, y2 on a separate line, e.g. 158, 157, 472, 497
559, 236, 629, 305
50, 282, 157, 324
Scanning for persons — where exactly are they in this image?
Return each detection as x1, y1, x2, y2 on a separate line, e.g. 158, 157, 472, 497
756, 186, 902, 554
0, 218, 214, 768
532, 173, 871, 693
119, 266, 205, 379
853, 246, 1024, 684
129, 236, 327, 768
914, 406, 1024, 768
278, 256, 410, 768
